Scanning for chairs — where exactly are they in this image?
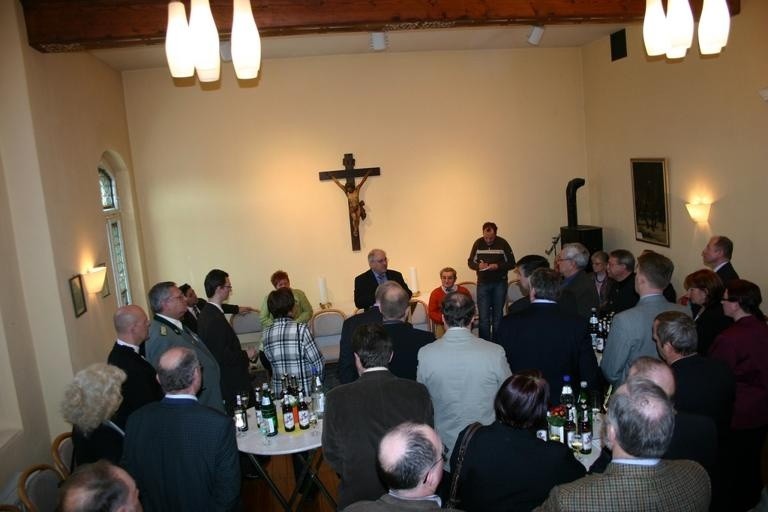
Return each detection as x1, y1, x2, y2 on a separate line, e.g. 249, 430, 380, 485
505, 281, 526, 312
14, 460, 66, 511
410, 300, 432, 335
458, 282, 485, 333
356, 308, 365, 314
311, 310, 348, 364
0, 502, 26, 510
228, 310, 271, 371
48, 427, 79, 480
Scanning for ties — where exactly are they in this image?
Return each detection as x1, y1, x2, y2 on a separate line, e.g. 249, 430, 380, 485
378, 275, 385, 284
193, 306, 199, 318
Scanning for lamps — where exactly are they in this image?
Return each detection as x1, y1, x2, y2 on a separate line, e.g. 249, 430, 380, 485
640, 1, 731, 60
84, 265, 110, 295
164, 0, 261, 82
681, 197, 712, 224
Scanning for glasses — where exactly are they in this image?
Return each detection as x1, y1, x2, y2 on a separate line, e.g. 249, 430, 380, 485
422, 443, 449, 484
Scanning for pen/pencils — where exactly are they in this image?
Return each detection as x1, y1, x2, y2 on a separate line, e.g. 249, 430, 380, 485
476, 260, 488, 268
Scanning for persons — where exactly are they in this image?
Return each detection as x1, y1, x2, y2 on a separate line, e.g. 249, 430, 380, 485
330, 172, 370, 237
468, 222, 516, 336
321, 248, 513, 512
60, 348, 240, 511
107, 269, 271, 478
444, 236, 768, 511
259, 270, 322, 492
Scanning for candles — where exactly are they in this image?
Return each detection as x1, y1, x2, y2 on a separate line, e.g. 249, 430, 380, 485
407, 266, 418, 293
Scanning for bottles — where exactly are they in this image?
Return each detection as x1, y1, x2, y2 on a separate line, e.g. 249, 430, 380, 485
588, 305, 615, 354
231, 372, 327, 437
537, 376, 600, 455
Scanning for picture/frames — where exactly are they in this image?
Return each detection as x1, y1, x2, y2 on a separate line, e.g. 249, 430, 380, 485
93, 262, 111, 301
68, 274, 88, 319
628, 156, 671, 248
